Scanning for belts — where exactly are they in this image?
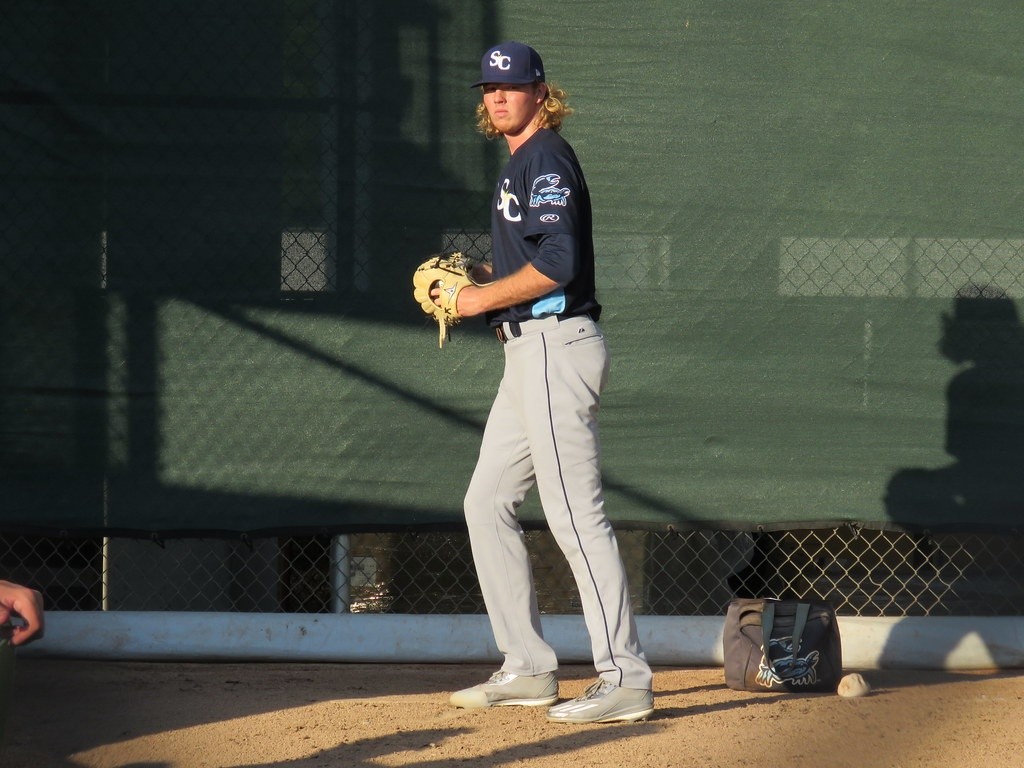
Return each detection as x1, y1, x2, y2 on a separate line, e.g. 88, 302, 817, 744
495, 314, 584, 343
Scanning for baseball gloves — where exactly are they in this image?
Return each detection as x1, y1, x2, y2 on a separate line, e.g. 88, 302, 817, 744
412, 250, 480, 329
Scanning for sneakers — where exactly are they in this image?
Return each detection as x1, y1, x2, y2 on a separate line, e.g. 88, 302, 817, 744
448, 673, 559, 707
546, 678, 654, 724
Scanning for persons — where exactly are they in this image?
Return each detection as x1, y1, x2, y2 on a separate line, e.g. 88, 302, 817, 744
412, 40, 654, 724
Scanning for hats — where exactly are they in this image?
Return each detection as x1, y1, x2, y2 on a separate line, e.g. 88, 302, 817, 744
470, 40, 545, 88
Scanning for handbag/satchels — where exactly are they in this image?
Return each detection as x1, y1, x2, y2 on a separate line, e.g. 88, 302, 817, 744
723, 598, 843, 692
